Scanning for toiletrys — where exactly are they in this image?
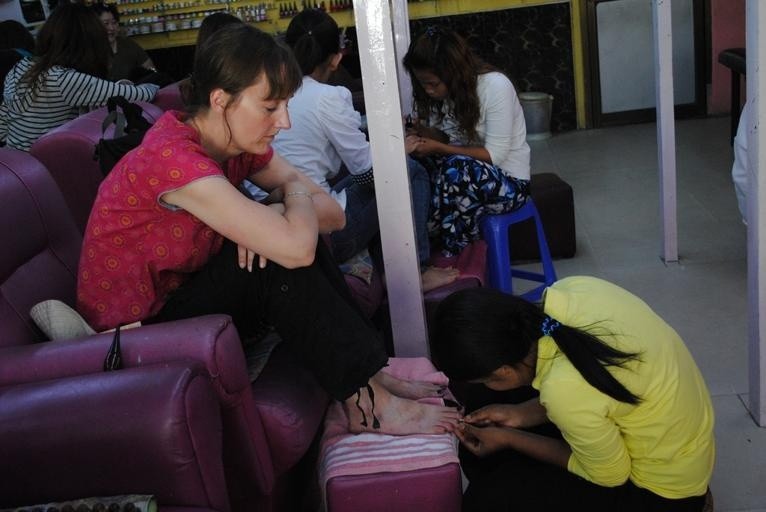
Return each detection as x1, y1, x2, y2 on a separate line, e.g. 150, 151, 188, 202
115, 1, 353, 37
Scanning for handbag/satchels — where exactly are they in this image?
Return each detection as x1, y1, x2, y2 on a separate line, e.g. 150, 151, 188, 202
93, 96, 153, 177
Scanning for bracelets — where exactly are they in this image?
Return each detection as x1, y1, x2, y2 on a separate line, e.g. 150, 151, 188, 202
283, 191, 312, 204
149, 66, 158, 73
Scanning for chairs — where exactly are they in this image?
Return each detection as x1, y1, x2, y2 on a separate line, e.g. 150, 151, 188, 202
0, 73, 380, 512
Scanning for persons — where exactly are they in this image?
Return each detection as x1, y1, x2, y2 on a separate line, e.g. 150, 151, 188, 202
91, 4, 158, 88
241, 7, 461, 295
402, 23, 532, 257
191, 11, 242, 71
0, 1, 161, 153
0, 19, 36, 104
431, 274, 716, 511
75, 23, 466, 436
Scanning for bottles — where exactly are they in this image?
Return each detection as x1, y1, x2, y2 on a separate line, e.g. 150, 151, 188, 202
117, 0, 353, 36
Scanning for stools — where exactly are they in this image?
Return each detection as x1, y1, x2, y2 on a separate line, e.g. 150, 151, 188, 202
424, 173, 577, 303
324, 398, 464, 512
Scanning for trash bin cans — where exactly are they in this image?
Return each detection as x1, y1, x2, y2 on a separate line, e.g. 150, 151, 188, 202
518, 92, 554, 141
